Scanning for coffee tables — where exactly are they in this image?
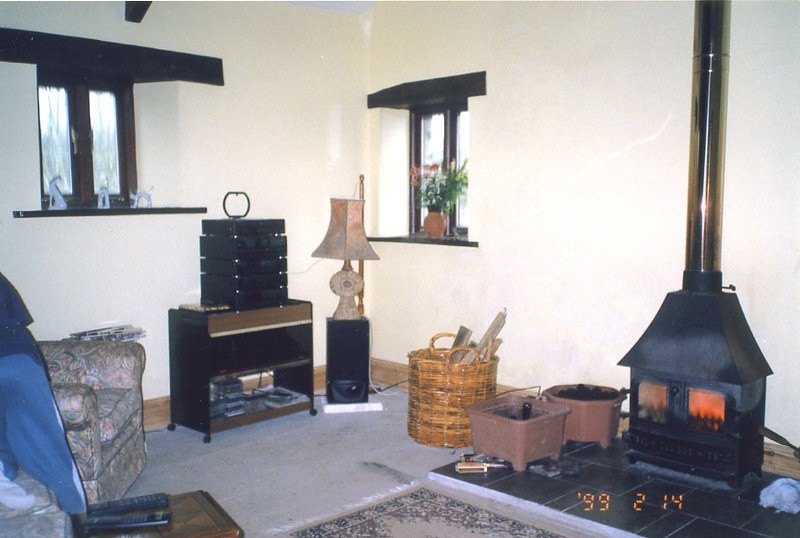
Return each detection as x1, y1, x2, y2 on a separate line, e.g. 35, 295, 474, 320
71, 490, 245, 538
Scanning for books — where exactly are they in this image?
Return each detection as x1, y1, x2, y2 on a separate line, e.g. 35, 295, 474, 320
62, 324, 146, 343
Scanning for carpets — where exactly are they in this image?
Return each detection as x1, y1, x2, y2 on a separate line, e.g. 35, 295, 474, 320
266, 482, 612, 538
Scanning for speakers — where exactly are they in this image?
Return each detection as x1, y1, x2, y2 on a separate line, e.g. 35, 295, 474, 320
326, 315, 372, 403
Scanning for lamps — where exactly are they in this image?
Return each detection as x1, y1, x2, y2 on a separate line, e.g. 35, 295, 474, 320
311, 197, 380, 319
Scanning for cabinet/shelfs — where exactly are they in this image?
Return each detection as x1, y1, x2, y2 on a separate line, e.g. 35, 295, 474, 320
167, 300, 317, 443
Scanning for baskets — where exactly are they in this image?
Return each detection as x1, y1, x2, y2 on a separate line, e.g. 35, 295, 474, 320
407, 336, 500, 447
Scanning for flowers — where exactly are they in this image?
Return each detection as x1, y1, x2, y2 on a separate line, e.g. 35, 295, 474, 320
410, 157, 470, 213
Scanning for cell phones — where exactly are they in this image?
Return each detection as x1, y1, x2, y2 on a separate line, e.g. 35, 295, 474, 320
454, 462, 488, 473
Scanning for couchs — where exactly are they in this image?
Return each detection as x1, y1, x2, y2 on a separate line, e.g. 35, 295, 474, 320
0, 341, 148, 538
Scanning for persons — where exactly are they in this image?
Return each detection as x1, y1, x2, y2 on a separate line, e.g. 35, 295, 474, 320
0, 273, 86, 516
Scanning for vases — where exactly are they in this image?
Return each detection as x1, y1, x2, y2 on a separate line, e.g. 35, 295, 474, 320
422, 206, 449, 238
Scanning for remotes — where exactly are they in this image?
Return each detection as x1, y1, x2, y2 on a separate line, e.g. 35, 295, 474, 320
87, 491, 168, 516
82, 510, 172, 530
